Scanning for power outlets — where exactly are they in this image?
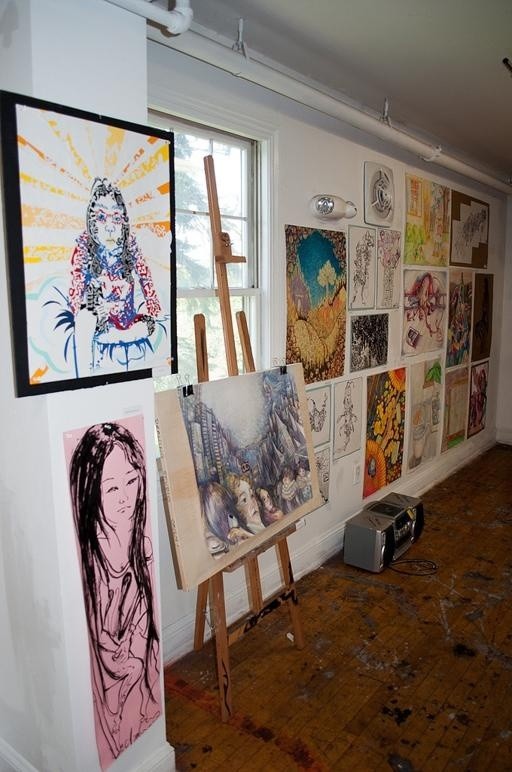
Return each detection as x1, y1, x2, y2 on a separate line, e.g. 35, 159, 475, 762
354, 464, 361, 484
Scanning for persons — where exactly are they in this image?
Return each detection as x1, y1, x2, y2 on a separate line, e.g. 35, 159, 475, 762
68, 423, 163, 770
202, 462, 312, 553
69, 176, 162, 329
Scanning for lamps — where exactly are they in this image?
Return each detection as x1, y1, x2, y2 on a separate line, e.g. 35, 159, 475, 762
307, 191, 360, 223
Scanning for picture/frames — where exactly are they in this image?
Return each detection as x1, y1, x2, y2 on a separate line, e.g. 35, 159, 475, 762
0, 90, 178, 398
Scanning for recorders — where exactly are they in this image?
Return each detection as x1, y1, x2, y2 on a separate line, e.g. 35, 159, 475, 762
343, 490, 424, 573
